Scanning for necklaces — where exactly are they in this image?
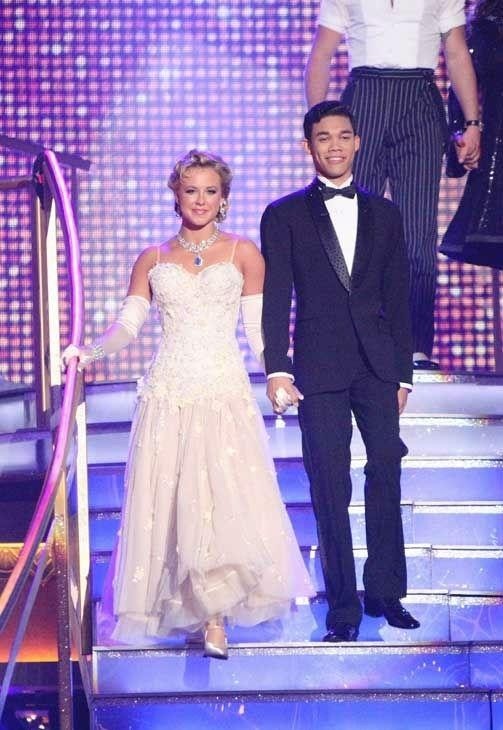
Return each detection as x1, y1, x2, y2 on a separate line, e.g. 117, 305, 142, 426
176, 228, 219, 267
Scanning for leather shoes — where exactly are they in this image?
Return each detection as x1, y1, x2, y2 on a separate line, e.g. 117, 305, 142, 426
412, 351, 439, 370
322, 622, 359, 641
363, 590, 420, 629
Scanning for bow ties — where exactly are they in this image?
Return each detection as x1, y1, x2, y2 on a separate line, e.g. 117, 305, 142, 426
321, 185, 356, 200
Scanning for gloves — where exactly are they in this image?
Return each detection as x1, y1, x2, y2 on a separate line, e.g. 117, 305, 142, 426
58, 295, 151, 374
241, 291, 305, 415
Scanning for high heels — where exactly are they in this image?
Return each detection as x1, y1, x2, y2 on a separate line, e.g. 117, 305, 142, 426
185, 624, 229, 660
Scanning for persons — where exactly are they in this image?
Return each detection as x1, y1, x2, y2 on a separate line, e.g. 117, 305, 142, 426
305, 0, 483, 372
60, 148, 316, 660
438, 0, 502, 273
258, 99, 420, 644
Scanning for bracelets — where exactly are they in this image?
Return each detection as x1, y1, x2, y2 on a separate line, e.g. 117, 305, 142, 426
87, 343, 105, 360
464, 119, 484, 132
